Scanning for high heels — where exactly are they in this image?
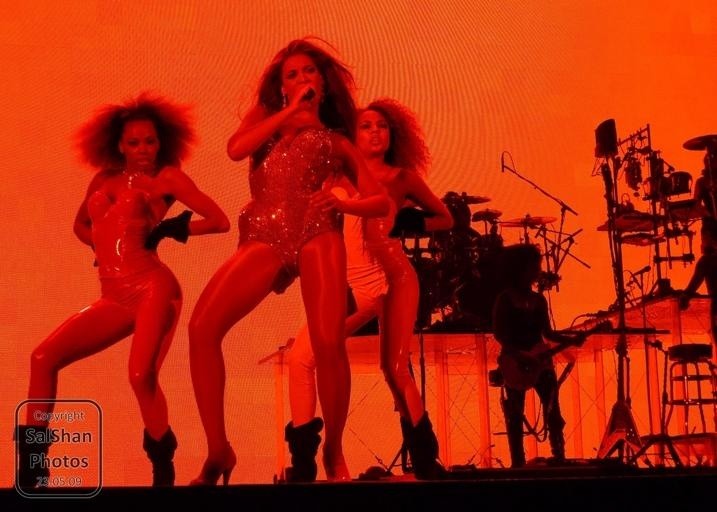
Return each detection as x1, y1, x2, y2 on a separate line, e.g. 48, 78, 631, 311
322, 438, 352, 484
187, 440, 242, 489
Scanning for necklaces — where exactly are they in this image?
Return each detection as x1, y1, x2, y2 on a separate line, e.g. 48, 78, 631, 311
123, 171, 140, 187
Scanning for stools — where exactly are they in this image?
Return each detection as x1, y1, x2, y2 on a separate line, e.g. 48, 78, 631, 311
659, 342, 716, 469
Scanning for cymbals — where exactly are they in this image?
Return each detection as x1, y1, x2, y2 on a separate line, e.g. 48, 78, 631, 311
500, 216, 557, 226
472, 210, 502, 222
460, 195, 490, 205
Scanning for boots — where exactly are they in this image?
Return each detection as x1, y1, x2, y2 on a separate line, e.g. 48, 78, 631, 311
397, 409, 451, 480
10, 422, 56, 491
500, 384, 530, 469
534, 377, 569, 462
279, 414, 326, 486
139, 426, 183, 500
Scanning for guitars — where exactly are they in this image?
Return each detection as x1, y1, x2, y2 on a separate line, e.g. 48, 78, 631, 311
489, 320, 616, 389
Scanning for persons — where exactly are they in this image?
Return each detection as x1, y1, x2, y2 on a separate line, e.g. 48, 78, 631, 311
679, 216, 716, 342
283, 97, 449, 483
14, 89, 230, 487
491, 243, 566, 468
187, 34, 389, 487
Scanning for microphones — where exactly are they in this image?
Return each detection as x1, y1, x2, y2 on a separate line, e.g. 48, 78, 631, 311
501, 156, 506, 173
